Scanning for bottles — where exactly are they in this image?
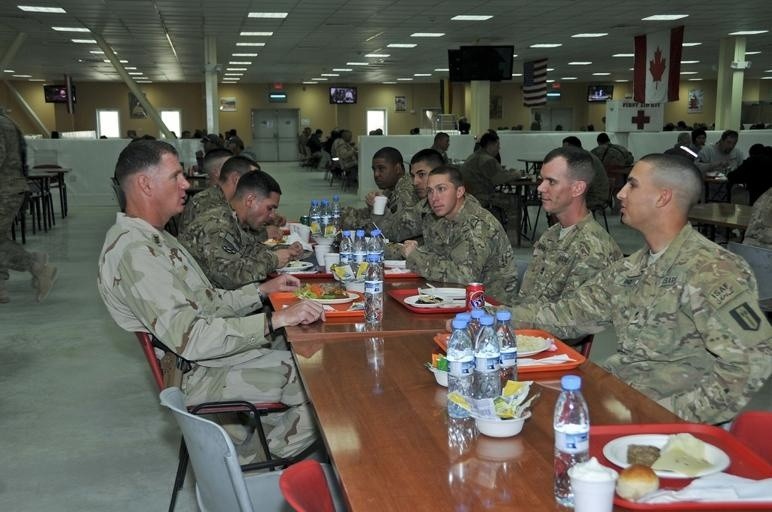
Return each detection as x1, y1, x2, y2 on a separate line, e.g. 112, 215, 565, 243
339, 229, 383, 325
552, 372, 593, 508
308, 195, 341, 234
447, 305, 517, 420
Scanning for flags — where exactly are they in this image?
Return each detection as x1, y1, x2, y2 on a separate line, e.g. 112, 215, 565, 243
522, 58, 548, 107
633, 24, 686, 104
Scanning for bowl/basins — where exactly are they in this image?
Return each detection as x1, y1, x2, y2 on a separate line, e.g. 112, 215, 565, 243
464, 410, 531, 437
424, 365, 475, 387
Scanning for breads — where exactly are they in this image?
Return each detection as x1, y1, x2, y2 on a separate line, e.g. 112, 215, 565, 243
617, 463, 658, 501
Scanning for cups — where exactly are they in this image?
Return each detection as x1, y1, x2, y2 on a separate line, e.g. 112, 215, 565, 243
566, 463, 620, 512
324, 252, 340, 274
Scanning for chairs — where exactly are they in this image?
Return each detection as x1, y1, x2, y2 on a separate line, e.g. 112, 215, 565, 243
565, 329, 598, 361
291, 139, 353, 190
134, 331, 313, 491
159, 381, 337, 512
728, 411, 772, 469
279, 459, 337, 511
11, 165, 71, 243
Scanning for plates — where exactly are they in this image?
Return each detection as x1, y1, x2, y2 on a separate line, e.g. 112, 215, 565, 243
262, 222, 551, 358
194, 173, 207, 177
295, 283, 361, 304
601, 432, 733, 479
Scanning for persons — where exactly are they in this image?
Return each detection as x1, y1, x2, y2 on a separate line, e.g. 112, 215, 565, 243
96, 139, 327, 468
1, 115, 60, 304
411, 114, 767, 247
298, 126, 382, 185
517, 148, 624, 354
345, 146, 518, 305
100, 127, 256, 160
178, 148, 304, 290
448, 152, 772, 424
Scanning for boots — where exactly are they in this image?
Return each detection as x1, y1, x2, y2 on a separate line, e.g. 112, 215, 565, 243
507, 230, 533, 249
30, 264, 58, 301
26, 251, 46, 289
0, 278, 12, 307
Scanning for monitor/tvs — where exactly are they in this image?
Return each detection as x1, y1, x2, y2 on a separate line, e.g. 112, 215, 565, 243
448, 45, 515, 80
587, 85, 614, 103
43, 84, 76, 103
329, 87, 357, 104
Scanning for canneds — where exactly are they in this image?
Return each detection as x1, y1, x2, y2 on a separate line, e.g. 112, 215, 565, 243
466, 282, 486, 311
